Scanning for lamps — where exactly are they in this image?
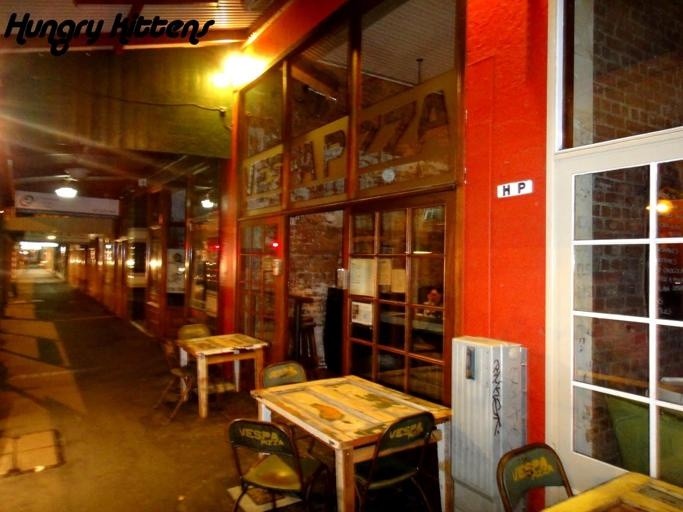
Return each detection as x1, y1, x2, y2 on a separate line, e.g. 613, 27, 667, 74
55, 187, 77, 199
643, 198, 674, 220
200, 189, 213, 209
17, 237, 61, 251
209, 53, 254, 88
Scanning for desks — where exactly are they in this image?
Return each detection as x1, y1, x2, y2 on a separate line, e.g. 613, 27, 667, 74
174, 333, 268, 421
367, 363, 445, 404
532, 472, 682, 511
249, 376, 448, 511
278, 292, 317, 364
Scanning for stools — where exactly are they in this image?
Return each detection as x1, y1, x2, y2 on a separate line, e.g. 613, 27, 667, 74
291, 315, 322, 366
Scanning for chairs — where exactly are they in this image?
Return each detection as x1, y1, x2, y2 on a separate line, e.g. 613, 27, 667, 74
222, 418, 328, 511
497, 441, 577, 512
341, 412, 437, 511
262, 361, 307, 388
152, 339, 219, 421
175, 324, 212, 337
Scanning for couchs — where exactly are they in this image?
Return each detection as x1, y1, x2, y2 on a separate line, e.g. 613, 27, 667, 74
604, 396, 680, 479
